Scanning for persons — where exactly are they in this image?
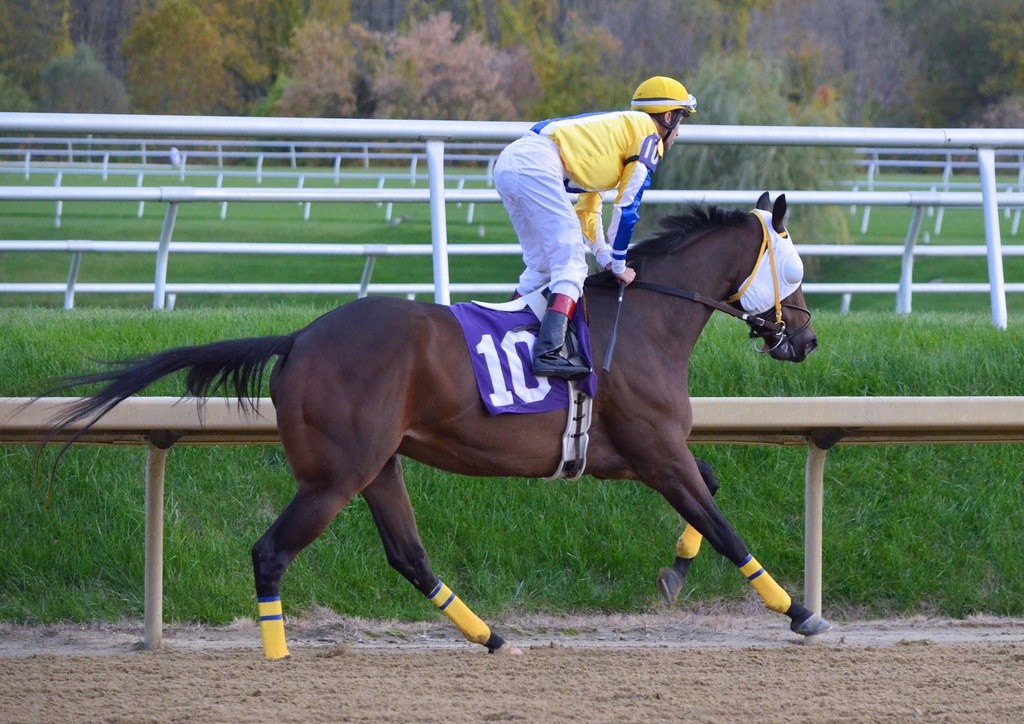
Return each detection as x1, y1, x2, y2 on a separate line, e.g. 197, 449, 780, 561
494, 76, 697, 378
170, 146, 181, 168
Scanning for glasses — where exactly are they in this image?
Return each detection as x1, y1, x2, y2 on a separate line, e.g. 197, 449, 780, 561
689, 93, 697, 110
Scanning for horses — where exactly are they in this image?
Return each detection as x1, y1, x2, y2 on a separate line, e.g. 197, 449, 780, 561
8, 192, 835, 662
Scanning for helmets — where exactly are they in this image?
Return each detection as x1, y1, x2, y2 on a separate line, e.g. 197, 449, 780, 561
631, 76, 697, 116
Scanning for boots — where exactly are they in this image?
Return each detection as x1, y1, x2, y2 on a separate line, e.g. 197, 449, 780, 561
533, 293, 590, 379
511, 288, 522, 301
